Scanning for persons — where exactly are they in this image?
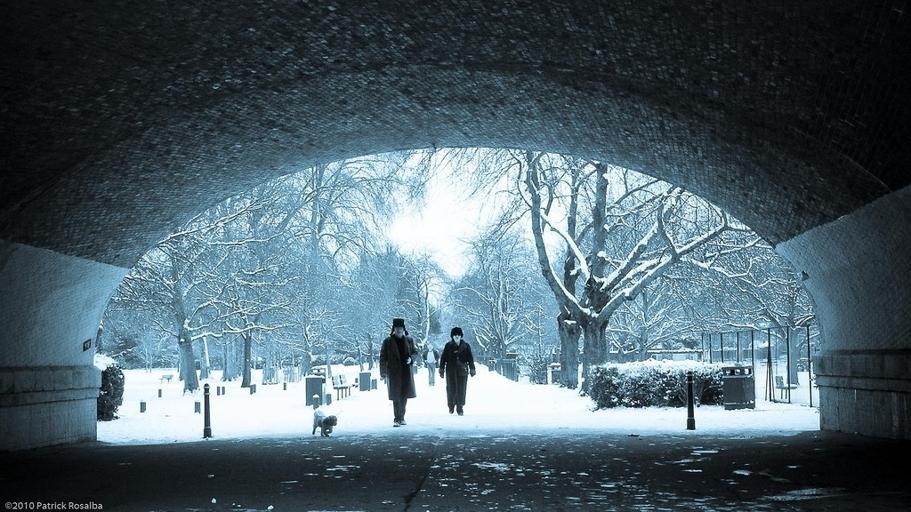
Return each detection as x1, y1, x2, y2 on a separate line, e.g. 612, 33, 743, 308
423, 341, 439, 387
439, 326, 477, 415
381, 318, 419, 426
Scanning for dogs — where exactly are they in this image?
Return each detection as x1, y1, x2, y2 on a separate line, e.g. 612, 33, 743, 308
312, 410, 337, 438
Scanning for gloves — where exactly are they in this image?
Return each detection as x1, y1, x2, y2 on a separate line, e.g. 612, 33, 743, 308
438, 368, 445, 378
470, 369, 476, 377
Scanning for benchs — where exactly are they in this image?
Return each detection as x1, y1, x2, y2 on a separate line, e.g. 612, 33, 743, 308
332, 374, 353, 400
776, 375, 797, 399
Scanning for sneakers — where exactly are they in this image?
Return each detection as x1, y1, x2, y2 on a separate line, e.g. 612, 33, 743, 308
449, 404, 466, 417
393, 418, 408, 427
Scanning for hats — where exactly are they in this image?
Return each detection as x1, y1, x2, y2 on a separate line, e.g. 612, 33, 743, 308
450, 326, 465, 338
390, 318, 409, 337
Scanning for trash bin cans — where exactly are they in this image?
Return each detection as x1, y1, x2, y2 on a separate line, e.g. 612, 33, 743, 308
306, 375, 322, 406
489, 360, 496, 371
360, 372, 371, 391
413, 366, 417, 374
720, 365, 755, 411
372, 379, 377, 390
548, 363, 562, 385
